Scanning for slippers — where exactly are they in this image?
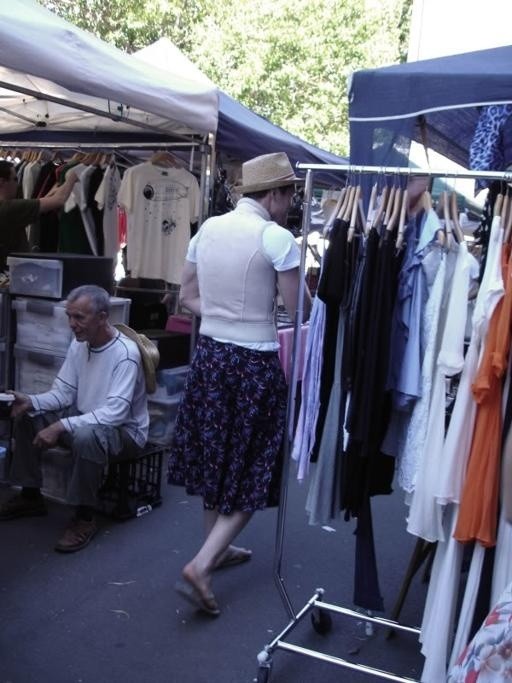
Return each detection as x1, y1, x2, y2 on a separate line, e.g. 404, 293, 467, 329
173, 580, 220, 615
209, 545, 252, 570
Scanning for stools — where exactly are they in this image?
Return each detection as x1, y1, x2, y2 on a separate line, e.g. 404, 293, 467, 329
39, 438, 174, 515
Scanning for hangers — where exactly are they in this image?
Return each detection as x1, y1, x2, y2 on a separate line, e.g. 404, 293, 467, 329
4, 138, 183, 175
318, 163, 511, 254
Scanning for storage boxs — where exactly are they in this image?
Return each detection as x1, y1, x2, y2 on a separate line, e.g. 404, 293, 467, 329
12, 291, 134, 356
6, 248, 118, 300
16, 347, 75, 416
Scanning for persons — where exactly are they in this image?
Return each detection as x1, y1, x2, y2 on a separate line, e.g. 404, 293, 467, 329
115, 243, 141, 302
0, 284, 150, 553
164, 151, 314, 618
2, 160, 79, 276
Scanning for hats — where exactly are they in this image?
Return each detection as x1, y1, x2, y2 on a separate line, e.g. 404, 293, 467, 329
112, 323, 160, 394
234, 151, 305, 194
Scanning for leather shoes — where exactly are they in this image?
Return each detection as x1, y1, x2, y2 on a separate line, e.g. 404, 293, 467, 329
54, 512, 99, 552
0, 492, 48, 522
